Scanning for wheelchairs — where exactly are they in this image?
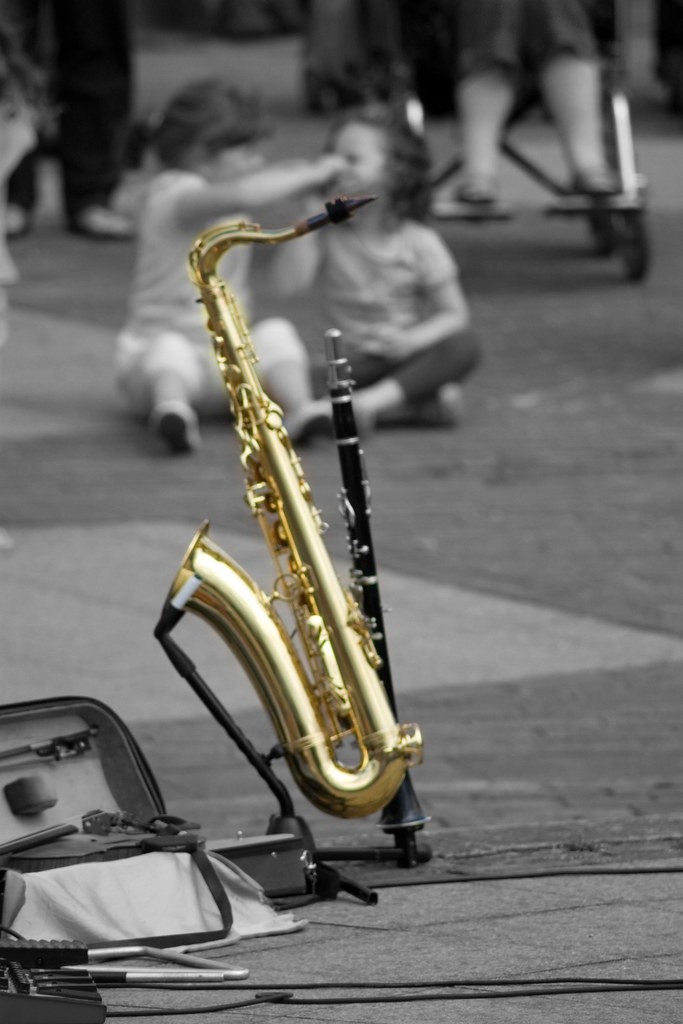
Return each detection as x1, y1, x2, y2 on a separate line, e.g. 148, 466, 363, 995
368, 1, 654, 290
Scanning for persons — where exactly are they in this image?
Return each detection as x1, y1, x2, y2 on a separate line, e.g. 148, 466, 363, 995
271, 101, 481, 424
116, 80, 347, 456
1, 0, 136, 235
451, 1, 622, 200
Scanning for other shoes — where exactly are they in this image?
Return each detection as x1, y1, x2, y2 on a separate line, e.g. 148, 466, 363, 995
574, 169, 644, 196
148, 404, 201, 453
4, 204, 32, 240
281, 407, 330, 447
68, 207, 136, 241
457, 180, 491, 203
421, 382, 464, 426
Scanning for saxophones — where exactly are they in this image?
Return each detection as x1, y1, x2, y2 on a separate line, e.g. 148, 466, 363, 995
164, 193, 426, 819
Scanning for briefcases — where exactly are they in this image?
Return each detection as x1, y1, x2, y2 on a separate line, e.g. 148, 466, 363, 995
0, 696, 316, 951
